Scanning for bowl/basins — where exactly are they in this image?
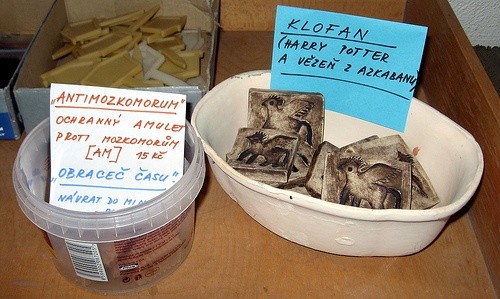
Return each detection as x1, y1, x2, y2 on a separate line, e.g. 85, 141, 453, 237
191, 70, 484, 256
11, 118, 205, 293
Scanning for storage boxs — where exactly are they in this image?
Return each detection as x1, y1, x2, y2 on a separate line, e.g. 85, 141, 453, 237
0, 0, 500, 299
